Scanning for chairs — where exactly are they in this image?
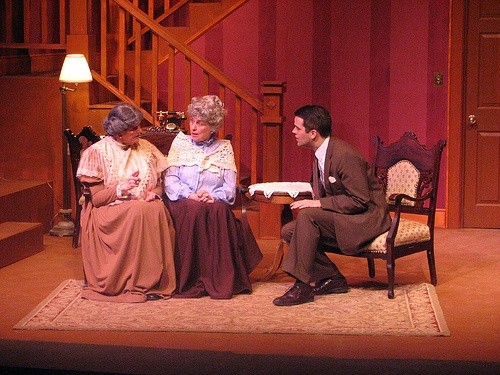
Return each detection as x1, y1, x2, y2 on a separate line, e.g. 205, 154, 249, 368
63, 126, 102, 248
324, 130, 447, 299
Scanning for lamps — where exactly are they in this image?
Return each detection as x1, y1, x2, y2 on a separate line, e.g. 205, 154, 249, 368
49, 54, 93, 236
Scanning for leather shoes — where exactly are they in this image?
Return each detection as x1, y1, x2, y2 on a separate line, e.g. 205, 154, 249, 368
312, 277, 347, 294
148, 294, 159, 300
273, 282, 314, 306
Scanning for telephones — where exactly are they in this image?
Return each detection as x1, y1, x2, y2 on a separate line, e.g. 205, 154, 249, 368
156, 110, 187, 133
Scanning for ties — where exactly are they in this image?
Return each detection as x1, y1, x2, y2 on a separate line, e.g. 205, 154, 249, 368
318, 159, 324, 188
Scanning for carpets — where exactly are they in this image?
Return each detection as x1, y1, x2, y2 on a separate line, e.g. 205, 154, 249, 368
12, 279, 451, 337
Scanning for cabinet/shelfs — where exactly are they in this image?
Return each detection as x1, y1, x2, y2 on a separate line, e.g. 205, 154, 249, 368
138, 130, 188, 158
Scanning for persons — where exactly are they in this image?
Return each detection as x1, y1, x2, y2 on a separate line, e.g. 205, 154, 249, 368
272, 104, 391, 306
162, 95, 262, 300
75, 103, 177, 303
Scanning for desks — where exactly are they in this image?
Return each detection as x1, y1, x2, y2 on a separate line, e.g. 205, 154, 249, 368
246, 182, 313, 281
